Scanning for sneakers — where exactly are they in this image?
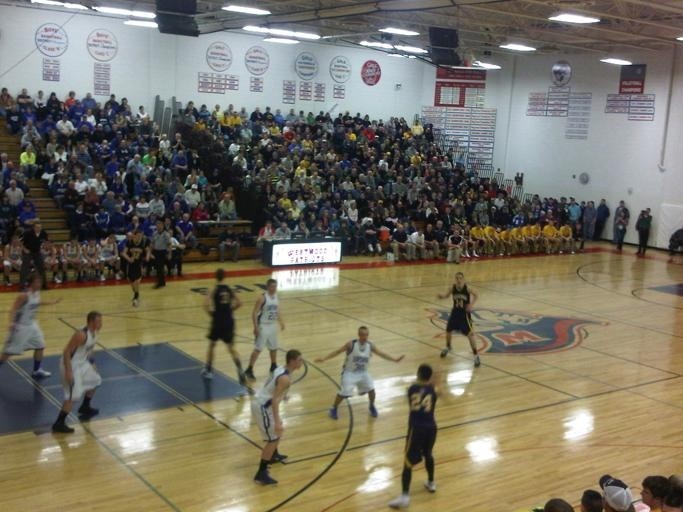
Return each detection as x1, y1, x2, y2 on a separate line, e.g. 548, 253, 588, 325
329, 407, 338, 420
474, 355, 480, 367
254, 473, 278, 484
32, 368, 51, 377
271, 455, 287, 464
52, 423, 74, 434
389, 497, 410, 508
201, 369, 213, 379
244, 370, 255, 380
79, 407, 98, 414
369, 405, 378, 417
441, 349, 448, 357
424, 482, 436, 493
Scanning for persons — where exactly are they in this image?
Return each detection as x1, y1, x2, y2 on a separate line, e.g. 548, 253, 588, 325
175, 213, 196, 241
447, 236, 461, 265
532, 498, 575, 511
544, 221, 559, 254
312, 219, 360, 255
462, 225, 480, 258
275, 222, 291, 240
39, 239, 62, 284
498, 228, 513, 257
244, 279, 286, 381
81, 238, 103, 283
597, 198, 609, 237
314, 326, 407, 420
250, 349, 303, 485
523, 224, 534, 252
257, 220, 275, 247
532, 223, 543, 254
615, 211, 629, 252
2, 235, 23, 287
435, 222, 447, 258
409, 228, 425, 259
200, 268, 248, 385
449, 226, 465, 259
99, 236, 121, 282
640, 475, 668, 512
483, 225, 498, 254
217, 227, 241, 263
613, 201, 630, 243
436, 271, 481, 367
166, 229, 188, 278
294, 222, 309, 239
1, 87, 149, 228
150, 101, 239, 221
361, 216, 385, 256
240, 107, 389, 219
120, 229, 151, 306
511, 226, 525, 255
0, 275, 62, 381
391, 224, 411, 262
51, 311, 105, 433
150, 220, 172, 289
559, 224, 575, 254
423, 224, 439, 260
389, 115, 585, 221
580, 490, 603, 512
19, 222, 51, 290
62, 238, 83, 284
599, 474, 635, 512
470, 223, 486, 256
663, 474, 683, 512
635, 207, 652, 257
583, 201, 597, 239
387, 363, 442, 509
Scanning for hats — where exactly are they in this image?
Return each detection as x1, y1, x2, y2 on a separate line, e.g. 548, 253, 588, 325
599, 474, 632, 512
